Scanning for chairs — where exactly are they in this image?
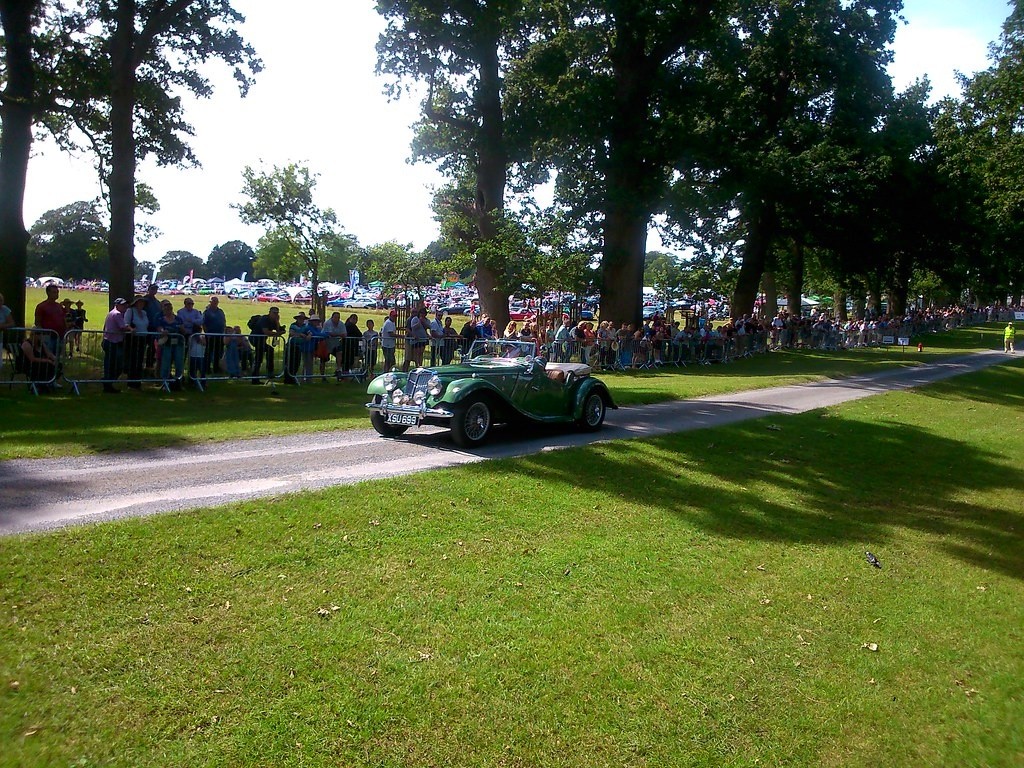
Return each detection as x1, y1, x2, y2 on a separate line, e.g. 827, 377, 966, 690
546, 369, 565, 383
6, 343, 32, 391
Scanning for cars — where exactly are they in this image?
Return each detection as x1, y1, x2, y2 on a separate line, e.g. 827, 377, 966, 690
366, 339, 618, 447
25, 275, 837, 325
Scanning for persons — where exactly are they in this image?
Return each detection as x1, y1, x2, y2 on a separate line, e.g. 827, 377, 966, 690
344, 313, 363, 374
202, 295, 227, 375
73, 300, 89, 352
379, 294, 1024, 375
362, 319, 379, 370
0, 293, 15, 368
284, 311, 310, 375
141, 283, 208, 392
222, 326, 242, 384
60, 298, 77, 359
100, 298, 130, 393
124, 295, 151, 390
305, 308, 322, 326
301, 314, 332, 382
233, 325, 254, 377
320, 311, 348, 383
28, 273, 106, 290
20, 325, 58, 393
248, 307, 286, 385
34, 284, 67, 388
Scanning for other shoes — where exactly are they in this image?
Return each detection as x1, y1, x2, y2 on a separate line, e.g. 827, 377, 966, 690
125, 378, 142, 391
46, 381, 63, 387
252, 378, 265, 385
101, 379, 120, 393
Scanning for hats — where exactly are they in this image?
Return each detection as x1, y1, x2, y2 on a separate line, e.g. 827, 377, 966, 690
75, 300, 84, 306
160, 299, 170, 306
147, 283, 159, 290
113, 297, 127, 305
611, 341, 620, 351
60, 297, 73, 304
129, 294, 146, 305
185, 298, 194, 304
389, 311, 397, 318
311, 314, 321, 322
293, 312, 309, 320
